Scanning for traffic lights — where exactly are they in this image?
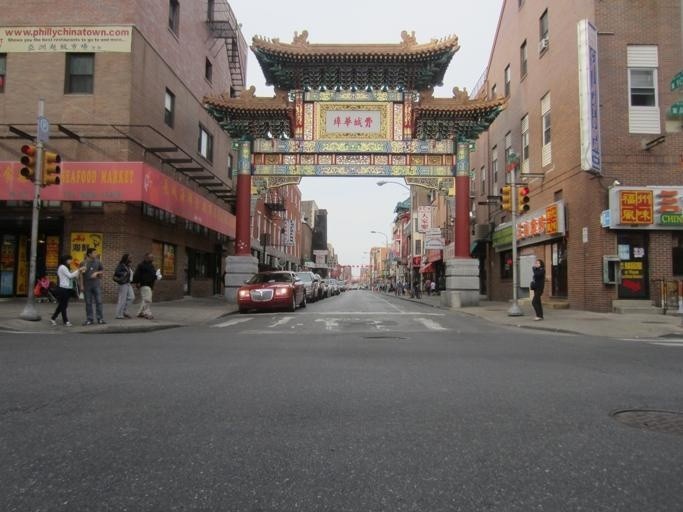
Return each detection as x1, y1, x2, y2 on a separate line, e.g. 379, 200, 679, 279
19, 144, 38, 181
500, 186, 511, 211
43, 153, 62, 185
517, 187, 529, 214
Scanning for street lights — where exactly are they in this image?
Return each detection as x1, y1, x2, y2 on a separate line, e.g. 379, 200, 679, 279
371, 231, 389, 260
370, 254, 423, 297
376, 181, 409, 192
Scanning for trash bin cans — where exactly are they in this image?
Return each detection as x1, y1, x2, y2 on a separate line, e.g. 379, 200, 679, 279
440, 291, 462, 308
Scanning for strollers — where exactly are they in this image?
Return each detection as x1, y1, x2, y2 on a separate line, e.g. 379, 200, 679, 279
36, 277, 57, 303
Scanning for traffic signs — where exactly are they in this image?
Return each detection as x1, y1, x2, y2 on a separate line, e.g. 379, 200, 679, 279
668, 70, 682, 92
667, 102, 683, 117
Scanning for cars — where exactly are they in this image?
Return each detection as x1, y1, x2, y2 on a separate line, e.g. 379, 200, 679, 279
236, 270, 367, 314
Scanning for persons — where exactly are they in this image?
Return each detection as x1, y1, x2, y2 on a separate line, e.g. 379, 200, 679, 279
531, 259, 545, 322
112, 254, 136, 320
35, 275, 51, 303
79, 248, 105, 326
50, 254, 87, 327
347, 274, 437, 300
134, 250, 162, 320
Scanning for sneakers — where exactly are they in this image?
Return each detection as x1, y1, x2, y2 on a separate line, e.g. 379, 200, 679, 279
49, 317, 56, 326
97, 318, 106, 325
82, 319, 93, 326
533, 316, 544, 321
63, 321, 74, 327
115, 311, 154, 320
